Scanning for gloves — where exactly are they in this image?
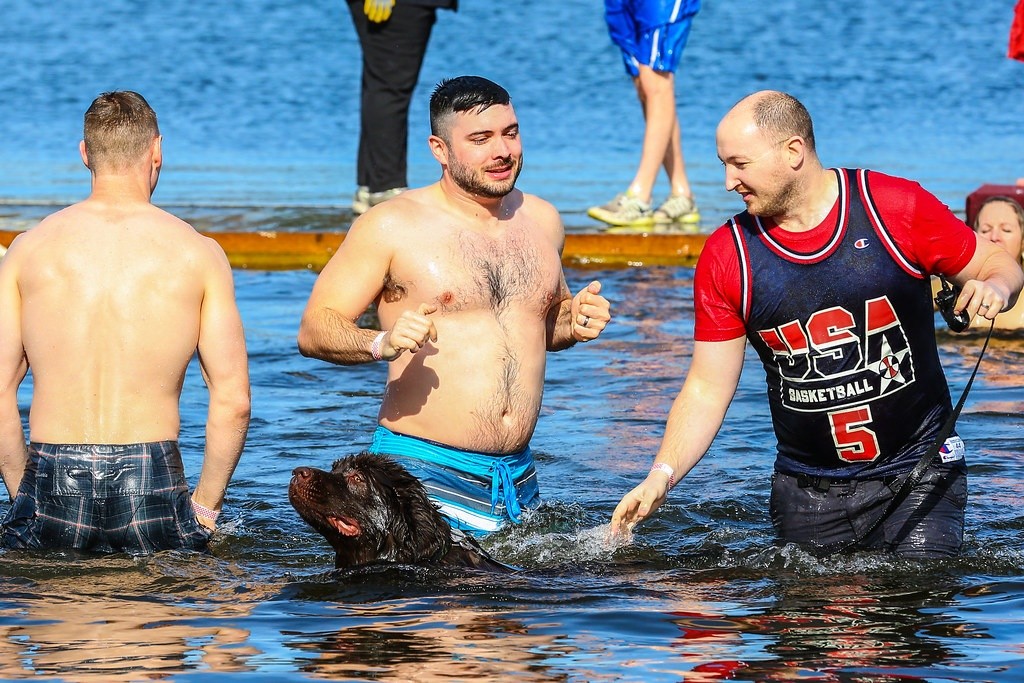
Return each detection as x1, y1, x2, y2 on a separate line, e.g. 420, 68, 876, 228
363, 0, 396, 23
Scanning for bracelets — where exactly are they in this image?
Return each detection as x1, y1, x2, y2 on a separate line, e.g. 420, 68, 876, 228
190, 498, 220, 522
372, 330, 389, 361
647, 463, 675, 490
981, 303, 989, 310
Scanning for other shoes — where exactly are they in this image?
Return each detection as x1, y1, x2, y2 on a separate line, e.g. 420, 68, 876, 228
351, 183, 408, 216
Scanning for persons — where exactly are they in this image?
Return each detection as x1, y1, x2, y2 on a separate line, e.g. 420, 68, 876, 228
0, 91, 252, 551
345, 0, 458, 214
931, 183, 1024, 329
587, 0, 701, 225
613, 89, 1024, 568
298, 76, 614, 538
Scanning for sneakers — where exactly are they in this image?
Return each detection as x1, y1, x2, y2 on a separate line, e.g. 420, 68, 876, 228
587, 195, 655, 227
654, 195, 699, 226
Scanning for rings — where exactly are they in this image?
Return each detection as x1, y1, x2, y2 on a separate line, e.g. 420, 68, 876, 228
583, 316, 589, 327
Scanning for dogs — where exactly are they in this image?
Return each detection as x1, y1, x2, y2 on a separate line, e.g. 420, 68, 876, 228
290, 452, 526, 575
